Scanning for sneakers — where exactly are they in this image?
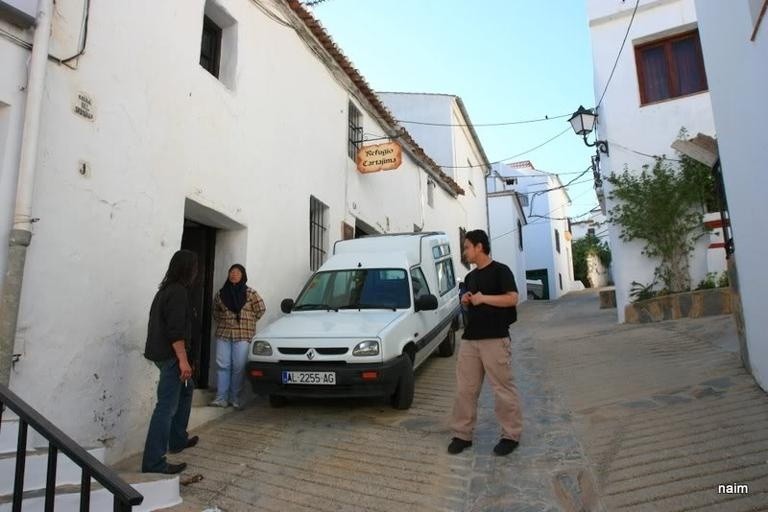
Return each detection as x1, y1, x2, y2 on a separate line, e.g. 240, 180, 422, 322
208, 400, 243, 410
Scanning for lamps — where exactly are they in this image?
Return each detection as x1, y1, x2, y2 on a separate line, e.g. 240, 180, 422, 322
566, 105, 609, 157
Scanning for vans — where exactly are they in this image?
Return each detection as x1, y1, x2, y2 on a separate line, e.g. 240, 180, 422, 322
249, 231, 463, 409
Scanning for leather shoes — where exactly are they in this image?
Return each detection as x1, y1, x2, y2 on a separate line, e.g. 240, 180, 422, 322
142, 456, 187, 473
167, 432, 199, 454
493, 439, 519, 455
448, 437, 472, 453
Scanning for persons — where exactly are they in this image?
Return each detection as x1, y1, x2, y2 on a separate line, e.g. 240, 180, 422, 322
141, 248, 200, 473
207, 263, 267, 411
446, 230, 524, 457
